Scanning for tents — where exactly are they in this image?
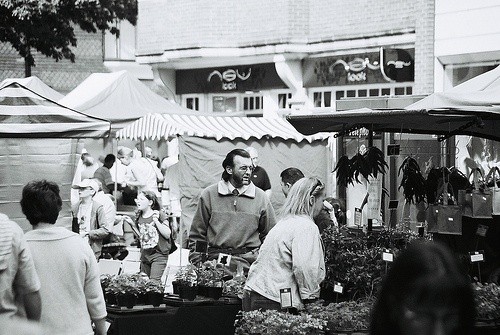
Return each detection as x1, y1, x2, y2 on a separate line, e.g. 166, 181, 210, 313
0, 62, 500, 247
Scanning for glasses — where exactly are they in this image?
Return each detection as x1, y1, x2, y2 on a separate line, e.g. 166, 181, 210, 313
232, 165, 255, 172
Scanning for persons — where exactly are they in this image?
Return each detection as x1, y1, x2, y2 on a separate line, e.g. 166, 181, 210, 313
0, 144, 475, 335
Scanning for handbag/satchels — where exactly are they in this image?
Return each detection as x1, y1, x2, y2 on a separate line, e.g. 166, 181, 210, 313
484, 166, 500, 215
427, 192, 462, 235
458, 168, 492, 218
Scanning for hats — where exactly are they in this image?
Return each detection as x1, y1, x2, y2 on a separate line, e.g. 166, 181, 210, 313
72, 178, 99, 192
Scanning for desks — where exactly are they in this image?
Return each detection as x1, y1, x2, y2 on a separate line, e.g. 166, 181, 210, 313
105, 294, 241, 335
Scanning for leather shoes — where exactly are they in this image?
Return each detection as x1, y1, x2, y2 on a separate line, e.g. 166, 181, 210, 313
209, 246, 257, 255
